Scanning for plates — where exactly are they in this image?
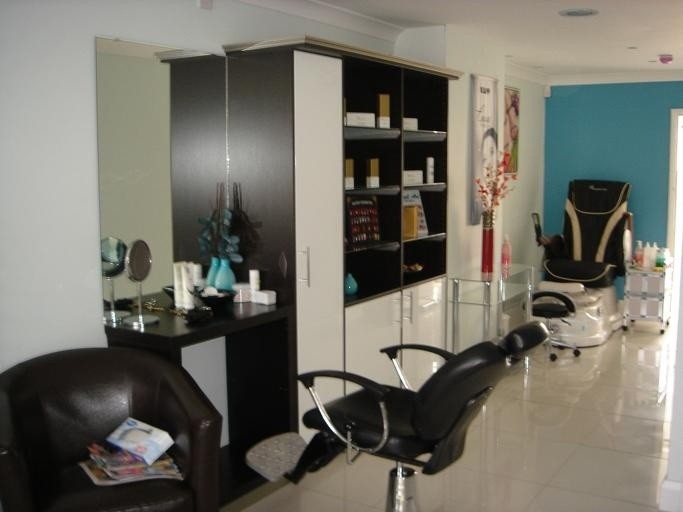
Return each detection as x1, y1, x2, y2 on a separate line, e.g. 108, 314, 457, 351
402, 263, 424, 274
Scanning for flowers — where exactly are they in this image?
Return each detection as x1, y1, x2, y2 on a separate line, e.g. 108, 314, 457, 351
474, 151, 521, 212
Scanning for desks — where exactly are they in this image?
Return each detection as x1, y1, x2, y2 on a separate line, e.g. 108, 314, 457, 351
103, 289, 300, 512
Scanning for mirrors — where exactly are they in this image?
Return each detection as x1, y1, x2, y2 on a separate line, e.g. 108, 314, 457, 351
119, 236, 163, 328
101, 235, 132, 325
94, 35, 228, 321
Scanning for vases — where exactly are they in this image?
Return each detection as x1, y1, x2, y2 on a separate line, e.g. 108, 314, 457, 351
480, 210, 495, 273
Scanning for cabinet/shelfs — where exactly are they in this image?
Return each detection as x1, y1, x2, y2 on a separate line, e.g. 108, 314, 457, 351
343, 45, 402, 310
621, 261, 674, 331
448, 262, 534, 355
403, 58, 462, 286
344, 274, 452, 397
225, 32, 342, 460
151, 48, 225, 280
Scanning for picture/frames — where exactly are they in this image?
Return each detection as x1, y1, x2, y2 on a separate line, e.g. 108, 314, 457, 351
467, 71, 499, 225
497, 84, 520, 176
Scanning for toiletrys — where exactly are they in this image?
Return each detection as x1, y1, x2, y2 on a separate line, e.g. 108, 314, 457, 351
171, 258, 276, 310
634, 239, 670, 272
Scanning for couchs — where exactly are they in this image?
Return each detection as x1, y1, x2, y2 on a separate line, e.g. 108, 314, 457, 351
0, 347, 223, 512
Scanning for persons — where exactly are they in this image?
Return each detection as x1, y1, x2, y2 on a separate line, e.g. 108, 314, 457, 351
480, 127, 496, 186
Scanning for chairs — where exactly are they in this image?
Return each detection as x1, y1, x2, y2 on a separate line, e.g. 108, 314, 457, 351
521, 289, 582, 364
243, 321, 552, 512
529, 178, 633, 349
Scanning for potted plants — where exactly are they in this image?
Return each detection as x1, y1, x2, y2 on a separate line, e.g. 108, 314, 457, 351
197, 216, 220, 289
214, 207, 243, 291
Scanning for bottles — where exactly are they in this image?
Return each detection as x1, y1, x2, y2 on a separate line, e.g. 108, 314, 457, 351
643, 241, 658, 268
247, 268, 261, 292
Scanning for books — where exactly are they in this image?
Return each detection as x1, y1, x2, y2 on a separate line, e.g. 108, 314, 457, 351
343, 91, 438, 246
79, 445, 182, 486
